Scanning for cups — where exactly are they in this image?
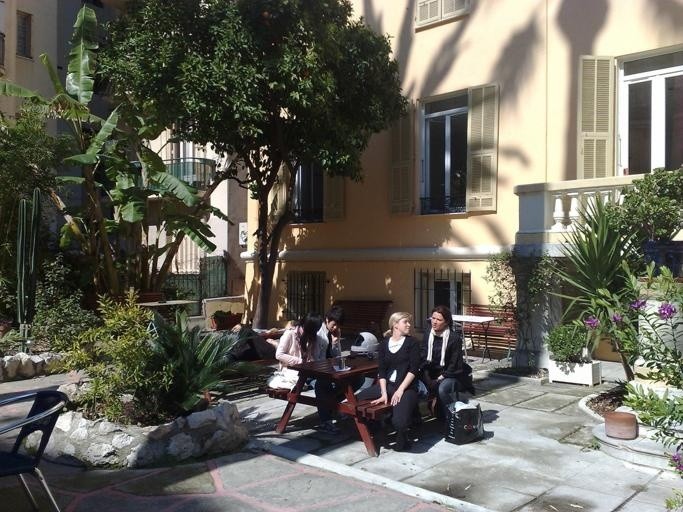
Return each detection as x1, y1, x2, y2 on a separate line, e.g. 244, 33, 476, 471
338, 358, 346, 370
368, 350, 373, 360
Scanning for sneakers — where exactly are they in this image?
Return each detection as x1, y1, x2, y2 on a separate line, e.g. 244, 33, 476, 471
319, 417, 410, 452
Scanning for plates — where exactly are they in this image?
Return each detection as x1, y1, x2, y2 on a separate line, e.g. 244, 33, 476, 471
332, 365, 351, 372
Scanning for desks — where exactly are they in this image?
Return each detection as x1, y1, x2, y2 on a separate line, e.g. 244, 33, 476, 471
427, 314, 495, 363
138, 300, 200, 334
276, 355, 435, 456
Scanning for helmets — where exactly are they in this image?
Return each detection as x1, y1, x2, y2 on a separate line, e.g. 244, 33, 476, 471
350, 331, 380, 353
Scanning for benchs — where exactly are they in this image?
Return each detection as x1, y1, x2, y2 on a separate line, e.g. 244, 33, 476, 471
460, 304, 519, 361
352, 399, 394, 420
265, 387, 313, 399
324, 300, 393, 346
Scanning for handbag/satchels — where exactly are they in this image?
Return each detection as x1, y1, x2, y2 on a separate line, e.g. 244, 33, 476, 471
444, 400, 486, 446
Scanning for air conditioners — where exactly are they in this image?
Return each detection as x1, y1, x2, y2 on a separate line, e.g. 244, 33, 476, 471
239, 222, 247, 249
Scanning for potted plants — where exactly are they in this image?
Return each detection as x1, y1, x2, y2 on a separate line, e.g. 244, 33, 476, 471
548, 323, 601, 387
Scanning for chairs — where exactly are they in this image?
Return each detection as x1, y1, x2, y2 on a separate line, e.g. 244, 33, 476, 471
0, 392, 68, 512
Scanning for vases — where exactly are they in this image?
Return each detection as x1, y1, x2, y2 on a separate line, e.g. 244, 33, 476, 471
605, 412, 637, 440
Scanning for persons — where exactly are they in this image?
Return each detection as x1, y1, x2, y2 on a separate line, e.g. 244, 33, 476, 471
354, 311, 421, 451
417, 305, 473, 437
267, 312, 342, 435
307, 305, 366, 404
